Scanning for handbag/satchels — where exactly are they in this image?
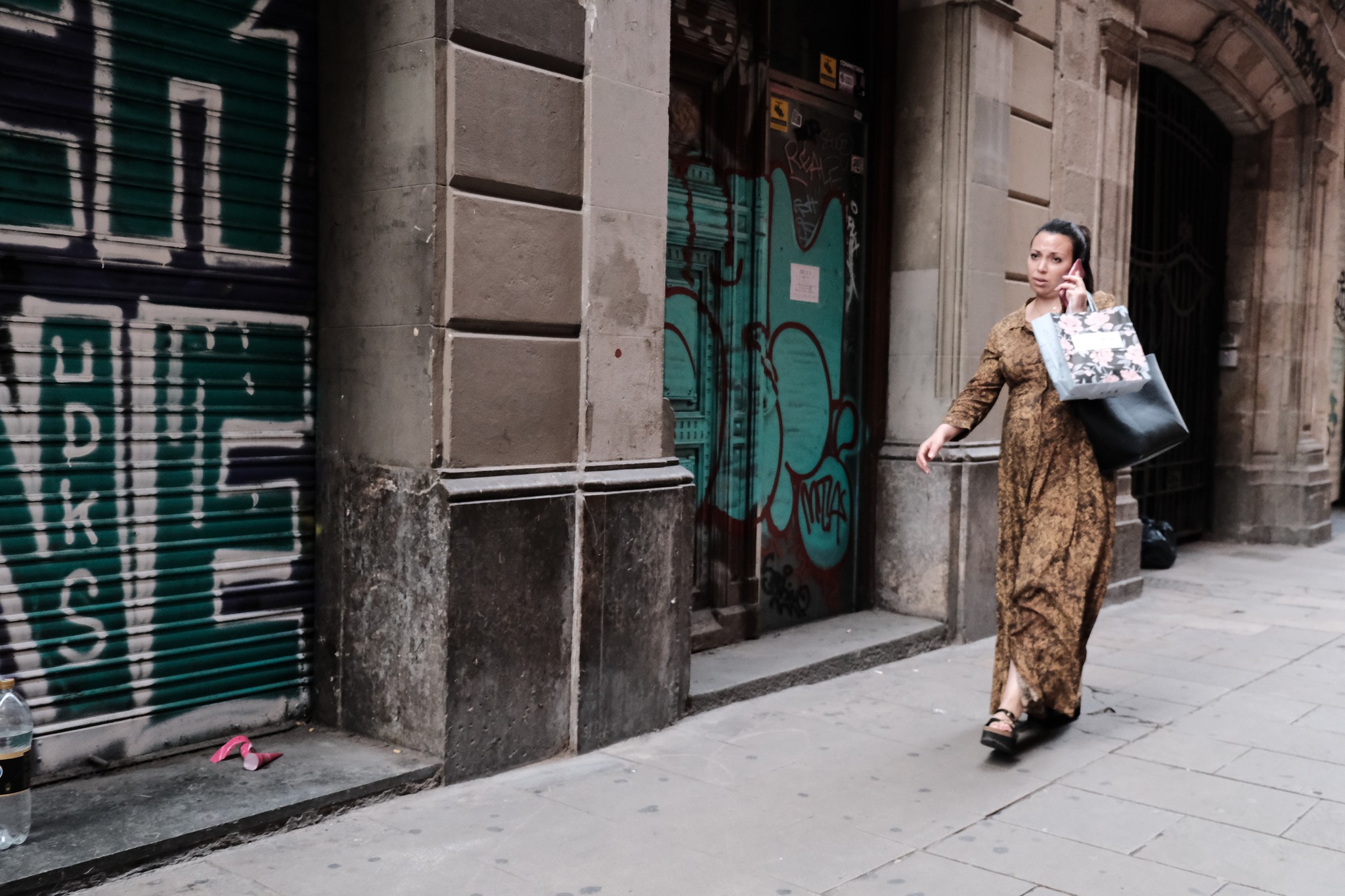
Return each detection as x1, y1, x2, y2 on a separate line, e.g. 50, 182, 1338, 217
1030, 289, 1151, 402
1077, 353, 1190, 478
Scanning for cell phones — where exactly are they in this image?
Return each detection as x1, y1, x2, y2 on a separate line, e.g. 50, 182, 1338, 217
1059, 259, 1085, 309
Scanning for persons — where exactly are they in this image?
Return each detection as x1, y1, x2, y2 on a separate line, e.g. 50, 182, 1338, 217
917, 217, 1123, 754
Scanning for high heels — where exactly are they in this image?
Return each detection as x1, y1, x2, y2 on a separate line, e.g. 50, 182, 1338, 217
1026, 699, 1082, 728
980, 709, 1020, 754
